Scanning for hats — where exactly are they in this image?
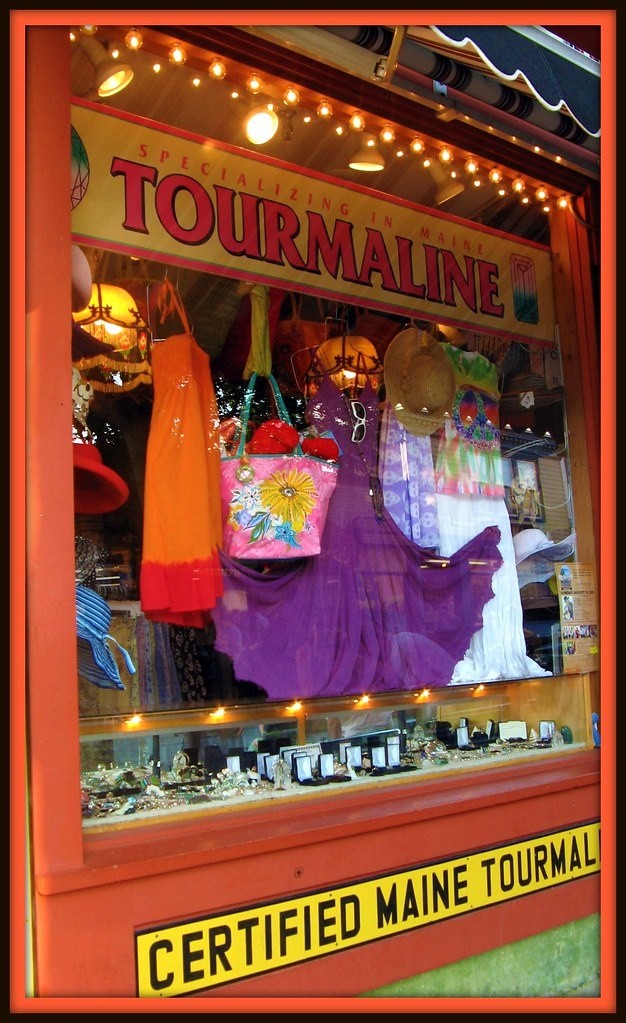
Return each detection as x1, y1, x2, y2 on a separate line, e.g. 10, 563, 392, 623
77, 583, 135, 691
384, 329, 457, 438
74, 439, 131, 514
513, 529, 576, 588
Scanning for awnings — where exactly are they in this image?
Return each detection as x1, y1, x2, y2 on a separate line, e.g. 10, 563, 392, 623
428, 26, 601, 138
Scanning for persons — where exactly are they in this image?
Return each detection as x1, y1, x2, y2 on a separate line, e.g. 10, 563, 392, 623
198, 726, 244, 760
248, 724, 297, 753
326, 711, 395, 739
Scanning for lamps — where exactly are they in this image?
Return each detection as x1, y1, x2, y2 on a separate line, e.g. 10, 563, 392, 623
424, 153, 468, 207
306, 335, 387, 394
345, 125, 386, 176
229, 92, 282, 146
73, 284, 146, 353
71, 28, 139, 101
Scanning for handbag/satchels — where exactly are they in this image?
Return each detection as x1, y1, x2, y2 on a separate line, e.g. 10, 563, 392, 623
221, 371, 340, 559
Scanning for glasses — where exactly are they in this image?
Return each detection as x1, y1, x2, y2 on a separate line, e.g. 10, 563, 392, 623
351, 402, 368, 443
